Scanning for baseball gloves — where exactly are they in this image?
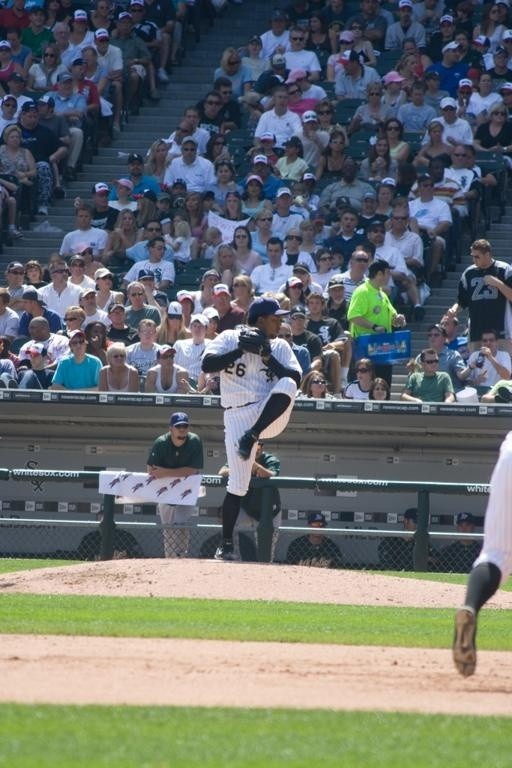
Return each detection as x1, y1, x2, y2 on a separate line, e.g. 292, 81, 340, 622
239, 326, 272, 359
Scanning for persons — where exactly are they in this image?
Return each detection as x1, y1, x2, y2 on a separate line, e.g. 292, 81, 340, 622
147, 411, 204, 558
76, 510, 144, 560
285, 512, 344, 569
199, 505, 257, 563
2, 0, 511, 406
375, 507, 438, 570
218, 441, 283, 562
448, 427, 512, 676
200, 295, 304, 560
432, 511, 484, 571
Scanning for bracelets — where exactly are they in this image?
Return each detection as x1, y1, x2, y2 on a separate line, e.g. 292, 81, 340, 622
460, 561, 502, 612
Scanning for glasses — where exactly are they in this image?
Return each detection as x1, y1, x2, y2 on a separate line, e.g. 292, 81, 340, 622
11, 272, 24, 276
353, 367, 368, 374
147, 226, 161, 235
427, 331, 442, 338
53, 267, 69, 276
426, 358, 440, 364
63, 316, 76, 322
130, 292, 144, 300
312, 378, 327, 385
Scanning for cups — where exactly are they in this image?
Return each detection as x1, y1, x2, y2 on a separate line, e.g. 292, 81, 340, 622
296, 195, 305, 206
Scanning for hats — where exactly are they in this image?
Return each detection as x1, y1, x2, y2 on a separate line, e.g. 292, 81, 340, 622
213, 284, 230, 298
456, 512, 476, 524
176, 291, 192, 304
0, 1, 148, 147
108, 303, 126, 311
307, 513, 329, 525
367, 259, 394, 271
79, 289, 95, 300
203, 270, 220, 279
93, 266, 112, 280
328, 277, 344, 289
246, 296, 290, 320
284, 277, 302, 288
7, 262, 25, 270
157, 344, 174, 359
201, 307, 218, 318
25, 342, 47, 357
68, 330, 85, 342
168, 412, 189, 425
139, 269, 154, 281
95, 0, 512, 252
168, 302, 181, 314
190, 312, 207, 328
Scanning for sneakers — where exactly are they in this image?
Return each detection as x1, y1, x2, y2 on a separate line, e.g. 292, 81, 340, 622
215, 541, 239, 561
236, 431, 254, 460
451, 607, 480, 679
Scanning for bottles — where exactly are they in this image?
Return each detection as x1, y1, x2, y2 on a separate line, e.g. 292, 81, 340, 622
476, 352, 485, 367
461, 98, 469, 117
